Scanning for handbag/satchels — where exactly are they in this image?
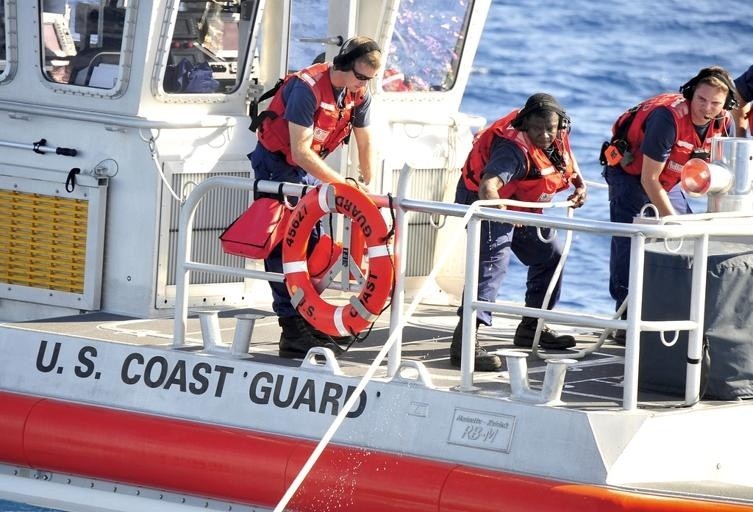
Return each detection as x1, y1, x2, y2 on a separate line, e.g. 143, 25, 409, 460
218, 198, 291, 259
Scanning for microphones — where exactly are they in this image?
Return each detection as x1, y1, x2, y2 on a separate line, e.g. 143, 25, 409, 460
704, 110, 732, 121
542, 122, 572, 152
360, 84, 370, 99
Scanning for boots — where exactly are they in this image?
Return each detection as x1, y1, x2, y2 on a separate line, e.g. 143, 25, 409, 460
449, 321, 502, 370
514, 317, 576, 348
278, 315, 352, 360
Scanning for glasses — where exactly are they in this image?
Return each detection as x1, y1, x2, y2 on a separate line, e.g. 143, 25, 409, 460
353, 69, 373, 80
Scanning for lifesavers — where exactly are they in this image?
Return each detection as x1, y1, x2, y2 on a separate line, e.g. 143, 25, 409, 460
282, 181, 393, 336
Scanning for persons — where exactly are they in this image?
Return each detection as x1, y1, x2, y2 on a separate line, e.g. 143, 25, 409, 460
600, 64, 752, 344
450, 94, 587, 371
246, 36, 383, 359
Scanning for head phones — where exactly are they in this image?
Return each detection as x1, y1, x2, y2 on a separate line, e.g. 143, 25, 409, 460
333, 41, 381, 71
511, 101, 571, 131
682, 71, 737, 110
